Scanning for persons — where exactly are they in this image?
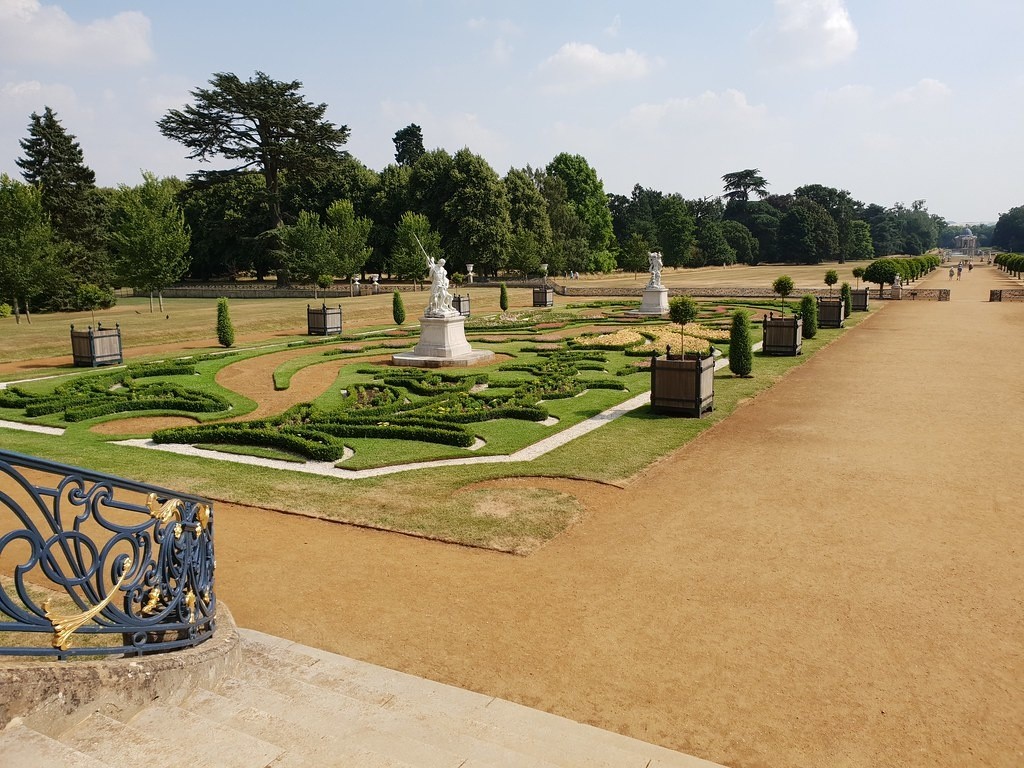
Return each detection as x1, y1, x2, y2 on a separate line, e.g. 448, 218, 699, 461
648, 251, 663, 286
575, 271, 578, 280
564, 271, 567, 282
948, 267, 955, 280
956, 261, 963, 281
570, 271, 573, 281
969, 262, 973, 272
426, 256, 456, 312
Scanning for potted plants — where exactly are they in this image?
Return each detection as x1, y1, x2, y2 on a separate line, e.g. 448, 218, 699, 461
818, 271, 845, 329
763, 275, 803, 357
533, 268, 553, 307
451, 272, 470, 318
307, 275, 343, 337
650, 295, 715, 419
70, 284, 123, 368
850, 266, 869, 311
